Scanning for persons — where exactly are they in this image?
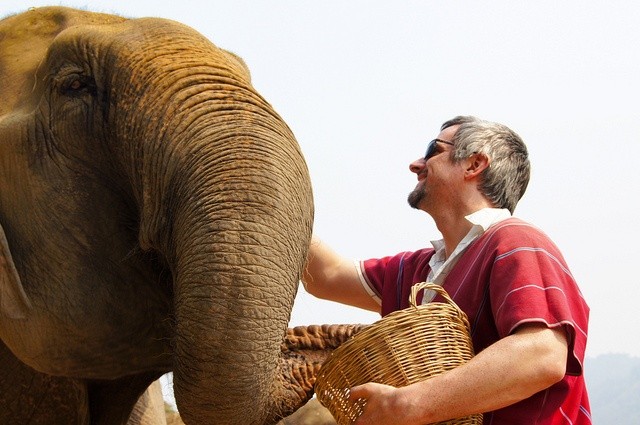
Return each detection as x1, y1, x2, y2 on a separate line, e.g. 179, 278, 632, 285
301, 114, 594, 424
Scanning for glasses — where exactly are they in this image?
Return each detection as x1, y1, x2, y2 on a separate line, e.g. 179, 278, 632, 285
424, 138, 478, 159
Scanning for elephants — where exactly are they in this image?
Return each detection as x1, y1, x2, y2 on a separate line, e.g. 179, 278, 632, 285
0, 7, 368, 425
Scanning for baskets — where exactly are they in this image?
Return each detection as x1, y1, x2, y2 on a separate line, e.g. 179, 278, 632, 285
313, 281, 484, 425
